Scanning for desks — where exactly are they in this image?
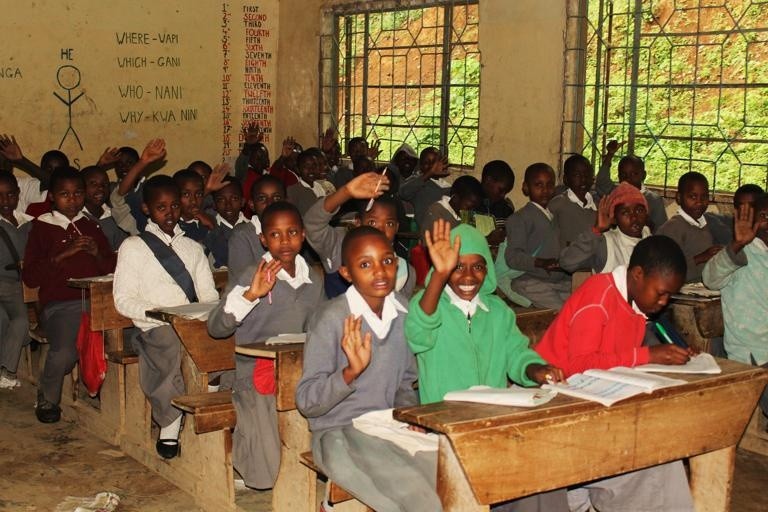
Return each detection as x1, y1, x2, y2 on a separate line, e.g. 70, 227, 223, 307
5, 237, 765, 512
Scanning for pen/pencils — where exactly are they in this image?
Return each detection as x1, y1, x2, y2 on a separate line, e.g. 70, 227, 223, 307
267, 267, 272, 304
656, 322, 673, 345
366, 166, 388, 211
72, 222, 82, 236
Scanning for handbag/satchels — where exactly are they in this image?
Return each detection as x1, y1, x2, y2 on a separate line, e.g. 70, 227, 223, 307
77, 313, 107, 392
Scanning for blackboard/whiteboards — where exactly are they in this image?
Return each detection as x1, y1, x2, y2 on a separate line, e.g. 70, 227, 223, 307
0, 0, 279, 180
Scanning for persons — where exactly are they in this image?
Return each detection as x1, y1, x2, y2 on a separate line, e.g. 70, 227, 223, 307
1, 123, 768, 512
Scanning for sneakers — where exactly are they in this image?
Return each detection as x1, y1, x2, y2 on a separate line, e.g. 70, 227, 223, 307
156, 414, 183, 458
37, 391, 60, 422
